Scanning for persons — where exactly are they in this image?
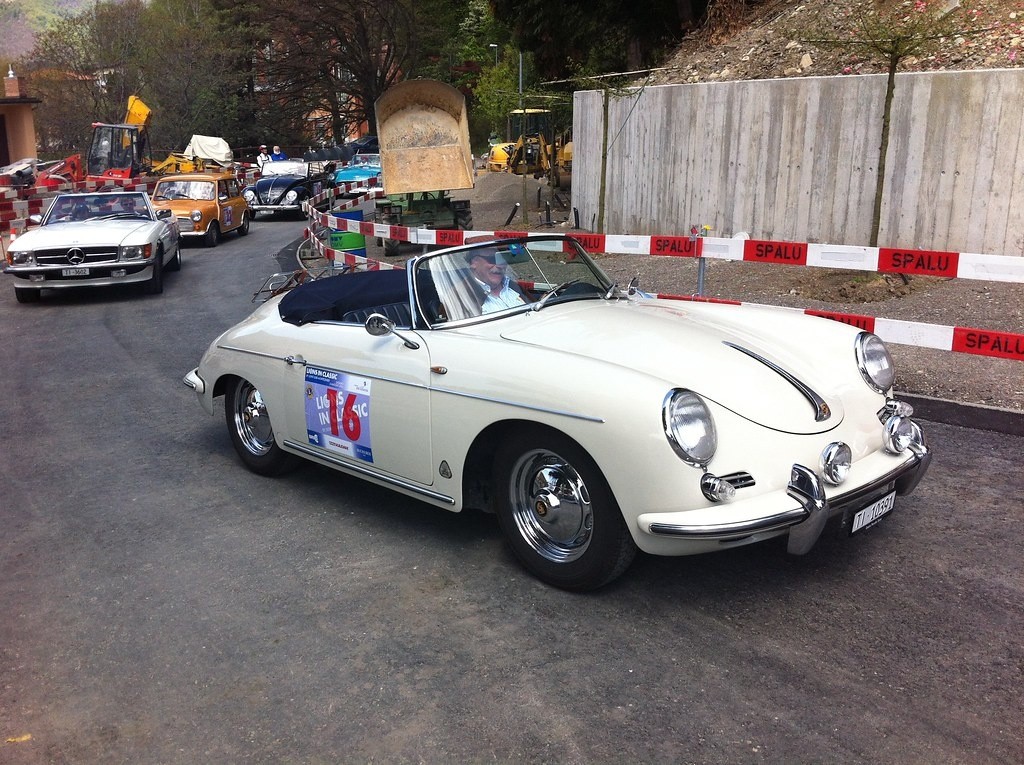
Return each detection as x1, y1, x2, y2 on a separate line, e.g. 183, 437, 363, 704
257, 145, 288, 178
72, 196, 148, 221
359, 156, 370, 165
435, 235, 538, 323
163, 181, 228, 201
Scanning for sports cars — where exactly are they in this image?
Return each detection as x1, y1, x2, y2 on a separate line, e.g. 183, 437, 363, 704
182, 232, 934, 594
241, 159, 338, 222
1, 192, 183, 303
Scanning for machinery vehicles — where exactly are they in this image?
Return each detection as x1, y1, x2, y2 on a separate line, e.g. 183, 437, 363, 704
487, 108, 572, 193
34, 95, 224, 190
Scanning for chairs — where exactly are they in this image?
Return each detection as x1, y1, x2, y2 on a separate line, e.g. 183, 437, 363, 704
424, 294, 440, 323
345, 302, 412, 327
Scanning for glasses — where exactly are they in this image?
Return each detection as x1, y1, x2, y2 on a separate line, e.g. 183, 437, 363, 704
472, 255, 496, 264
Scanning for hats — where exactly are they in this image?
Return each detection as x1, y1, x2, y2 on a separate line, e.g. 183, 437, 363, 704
465, 235, 508, 249
259, 145, 267, 150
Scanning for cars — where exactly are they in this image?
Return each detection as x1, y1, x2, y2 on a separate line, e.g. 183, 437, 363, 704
334, 153, 382, 198
145, 172, 250, 248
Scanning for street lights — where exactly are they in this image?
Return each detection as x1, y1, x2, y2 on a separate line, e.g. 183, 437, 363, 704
490, 43, 498, 74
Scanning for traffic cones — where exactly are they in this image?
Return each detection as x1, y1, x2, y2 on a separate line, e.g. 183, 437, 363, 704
7, 227, 17, 259
